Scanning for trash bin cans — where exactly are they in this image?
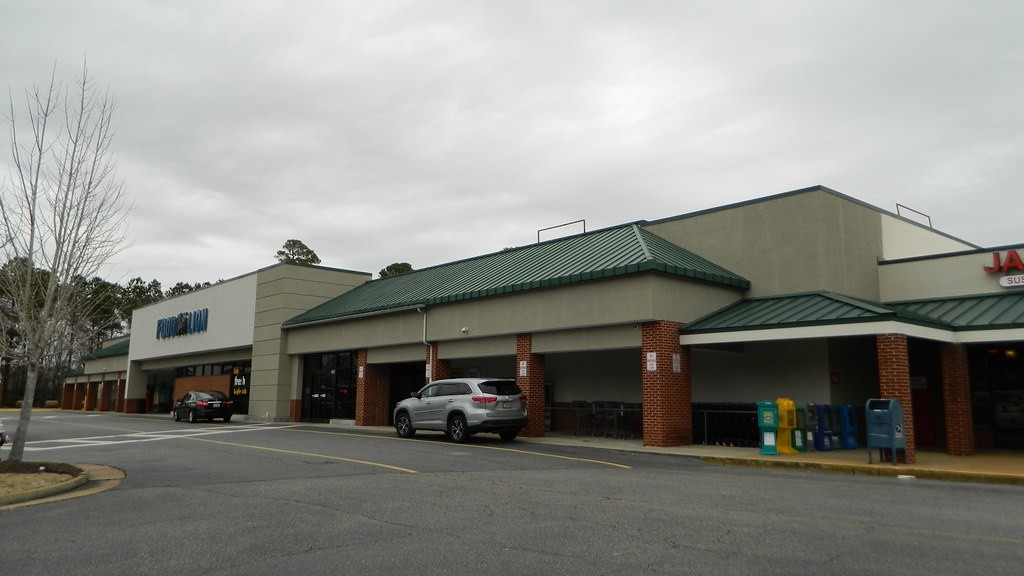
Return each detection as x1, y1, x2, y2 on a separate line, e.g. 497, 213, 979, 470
864, 400, 906, 466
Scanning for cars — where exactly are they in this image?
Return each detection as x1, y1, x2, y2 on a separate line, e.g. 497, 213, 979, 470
173, 390, 235, 424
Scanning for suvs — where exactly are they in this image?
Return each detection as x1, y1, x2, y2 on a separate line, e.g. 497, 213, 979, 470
393, 378, 528, 444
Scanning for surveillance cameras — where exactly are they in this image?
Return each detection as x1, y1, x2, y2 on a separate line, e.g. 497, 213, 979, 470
461, 327, 469, 334
